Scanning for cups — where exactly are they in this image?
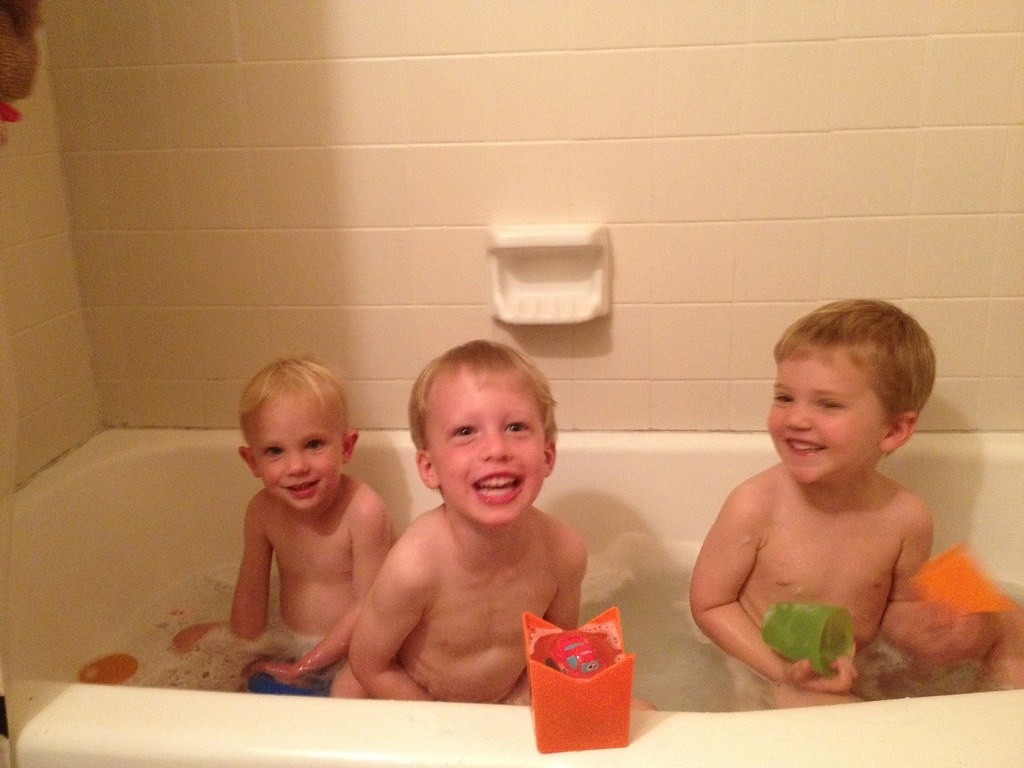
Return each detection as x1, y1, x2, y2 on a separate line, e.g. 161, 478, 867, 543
761, 602, 855, 677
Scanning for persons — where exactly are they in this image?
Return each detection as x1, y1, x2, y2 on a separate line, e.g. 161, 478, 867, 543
689, 299, 1024, 699
348, 339, 656, 710
228, 356, 400, 697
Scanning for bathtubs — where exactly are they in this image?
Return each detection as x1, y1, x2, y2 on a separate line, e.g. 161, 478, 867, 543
0, 424, 1020, 765
